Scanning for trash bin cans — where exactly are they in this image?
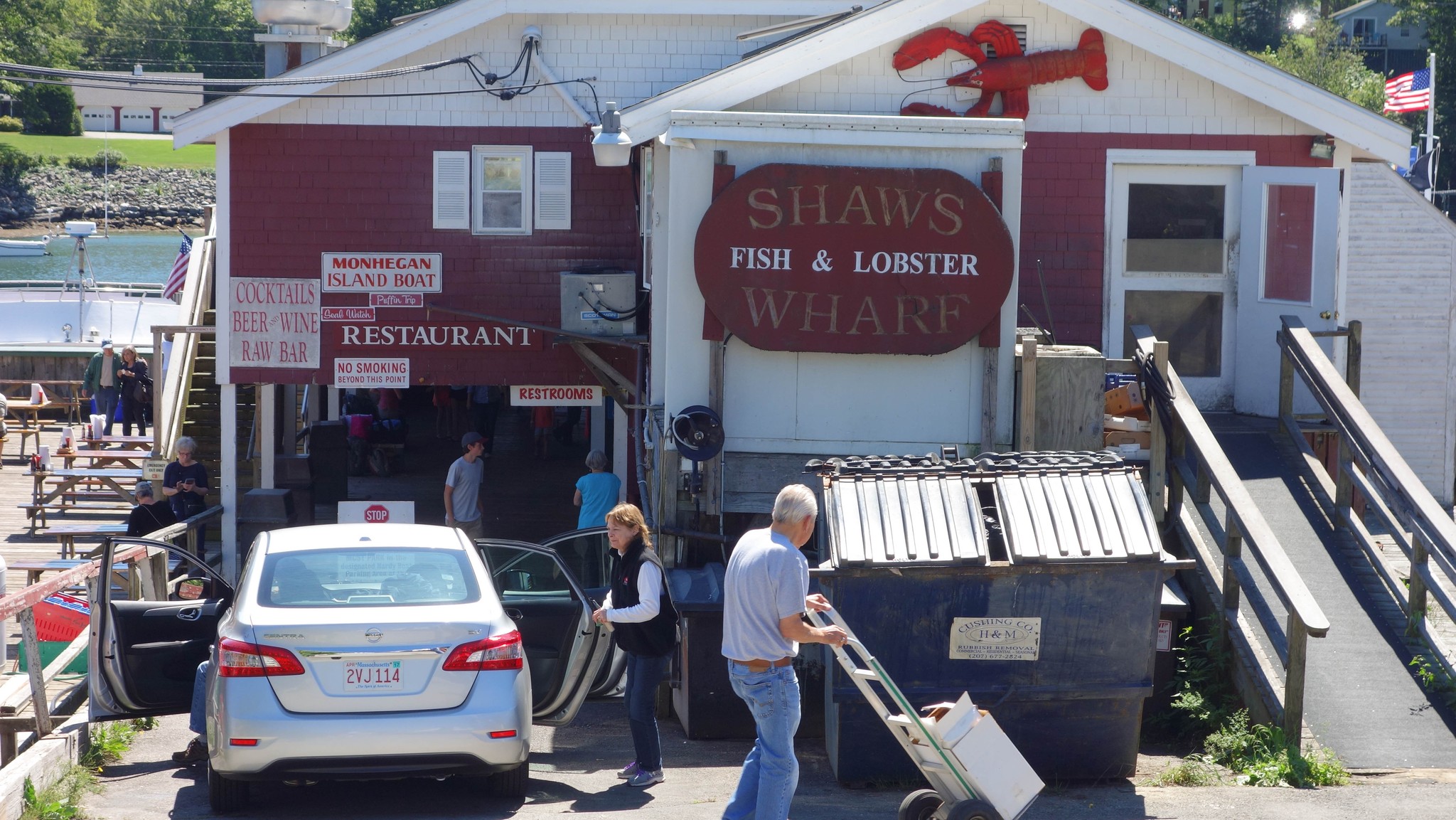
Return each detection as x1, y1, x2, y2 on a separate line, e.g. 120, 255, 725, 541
664, 561, 758, 740
91, 394, 123, 423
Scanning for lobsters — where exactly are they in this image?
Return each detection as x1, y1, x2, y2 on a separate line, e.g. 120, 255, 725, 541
892, 20, 1109, 121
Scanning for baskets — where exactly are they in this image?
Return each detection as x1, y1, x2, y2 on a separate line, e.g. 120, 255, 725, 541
30, 593, 88, 642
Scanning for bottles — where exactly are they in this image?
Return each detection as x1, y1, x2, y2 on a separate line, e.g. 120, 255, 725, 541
31, 453, 36, 472
39, 391, 43, 403
42, 463, 46, 471
50, 463, 53, 471
65, 437, 70, 448
63, 440, 66, 448
36, 454, 42, 471
82, 422, 85, 438
88, 424, 93, 437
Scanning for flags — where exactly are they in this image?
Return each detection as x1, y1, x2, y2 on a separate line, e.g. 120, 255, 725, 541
1382, 69, 1430, 115
163, 228, 193, 300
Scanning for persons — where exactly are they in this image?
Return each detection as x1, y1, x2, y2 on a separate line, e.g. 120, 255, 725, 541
163, 436, 208, 563
126, 481, 183, 561
343, 383, 592, 475
81, 338, 121, 446
117, 346, 148, 446
573, 449, 621, 589
592, 503, 678, 787
172, 660, 209, 763
442, 432, 483, 557
161, 333, 174, 390
720, 484, 848, 820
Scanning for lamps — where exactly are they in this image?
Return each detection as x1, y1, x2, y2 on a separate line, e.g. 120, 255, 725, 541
1309, 134, 1336, 160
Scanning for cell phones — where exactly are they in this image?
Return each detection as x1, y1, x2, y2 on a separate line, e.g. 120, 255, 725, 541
184, 478, 194, 485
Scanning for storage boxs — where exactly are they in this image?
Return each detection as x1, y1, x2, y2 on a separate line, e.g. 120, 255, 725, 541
898, 690, 1046, 820
1104, 370, 1152, 461
17, 592, 91, 674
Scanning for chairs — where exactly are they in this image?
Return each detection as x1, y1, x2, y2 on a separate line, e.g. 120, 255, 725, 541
279, 570, 322, 601
378, 573, 434, 602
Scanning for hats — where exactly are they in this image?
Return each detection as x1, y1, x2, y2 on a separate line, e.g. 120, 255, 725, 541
101, 338, 113, 349
462, 432, 490, 447
134, 481, 153, 501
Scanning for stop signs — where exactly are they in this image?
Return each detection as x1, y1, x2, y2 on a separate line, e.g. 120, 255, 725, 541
364, 504, 390, 523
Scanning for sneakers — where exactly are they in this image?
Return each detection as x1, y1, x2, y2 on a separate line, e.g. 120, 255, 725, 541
626, 766, 667, 787
617, 759, 642, 779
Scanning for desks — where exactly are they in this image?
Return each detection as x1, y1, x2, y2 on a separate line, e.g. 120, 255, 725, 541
49, 450, 151, 515
75, 435, 154, 493
22, 469, 143, 537
6, 560, 180, 623
0, 380, 85, 426
43, 523, 128, 560
5, 400, 52, 463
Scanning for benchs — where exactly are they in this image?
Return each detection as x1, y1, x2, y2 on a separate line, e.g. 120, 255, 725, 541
0, 395, 130, 597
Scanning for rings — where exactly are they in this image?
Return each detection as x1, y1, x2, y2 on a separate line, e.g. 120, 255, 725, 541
598, 616, 602, 621
840, 637, 845, 643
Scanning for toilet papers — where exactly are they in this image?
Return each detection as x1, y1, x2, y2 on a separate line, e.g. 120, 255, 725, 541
39, 445, 51, 470
30, 383, 48, 404
89, 414, 106, 438
62, 427, 78, 451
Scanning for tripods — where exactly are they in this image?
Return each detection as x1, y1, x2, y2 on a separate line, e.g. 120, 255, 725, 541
59, 238, 101, 342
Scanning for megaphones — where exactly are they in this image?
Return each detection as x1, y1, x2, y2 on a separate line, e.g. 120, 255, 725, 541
88, 325, 100, 337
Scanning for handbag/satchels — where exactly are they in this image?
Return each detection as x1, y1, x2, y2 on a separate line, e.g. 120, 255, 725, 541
133, 378, 153, 403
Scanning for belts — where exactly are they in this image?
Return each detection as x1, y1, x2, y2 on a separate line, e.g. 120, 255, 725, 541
730, 657, 793, 668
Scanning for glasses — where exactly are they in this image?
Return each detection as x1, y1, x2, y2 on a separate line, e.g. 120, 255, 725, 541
178, 451, 192, 456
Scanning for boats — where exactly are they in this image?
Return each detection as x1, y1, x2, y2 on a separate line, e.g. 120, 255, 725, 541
0, 220, 183, 349
0, 236, 51, 257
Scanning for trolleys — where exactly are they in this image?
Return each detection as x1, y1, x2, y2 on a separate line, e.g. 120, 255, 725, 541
804, 601, 1000, 820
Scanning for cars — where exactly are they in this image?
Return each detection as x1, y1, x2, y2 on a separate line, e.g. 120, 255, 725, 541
86, 522, 636, 814
